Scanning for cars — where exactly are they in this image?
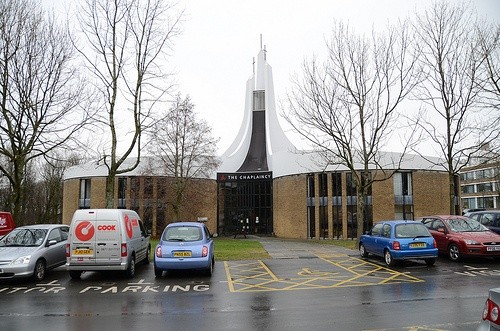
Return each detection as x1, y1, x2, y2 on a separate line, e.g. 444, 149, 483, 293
416, 207, 500, 262
154, 222, 215, 277
358, 219, 439, 266
0, 211, 70, 281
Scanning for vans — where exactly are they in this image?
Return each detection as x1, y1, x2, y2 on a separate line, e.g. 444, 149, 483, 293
65, 208, 151, 279
